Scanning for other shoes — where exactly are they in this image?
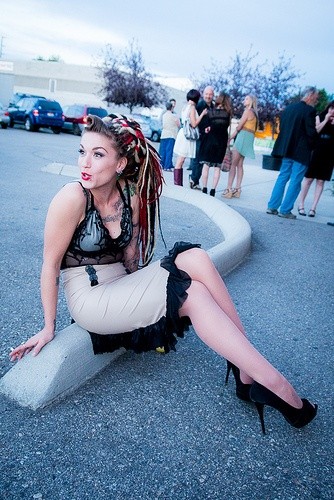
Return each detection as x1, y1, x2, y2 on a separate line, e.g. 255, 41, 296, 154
265, 208, 279, 215
202, 187, 208, 194
189, 181, 194, 189
308, 209, 315, 217
186, 167, 192, 170
193, 185, 201, 190
277, 211, 297, 219
210, 189, 216, 197
164, 169, 173, 172
298, 205, 307, 216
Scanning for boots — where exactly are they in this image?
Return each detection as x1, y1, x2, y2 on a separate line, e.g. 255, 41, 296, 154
174, 168, 183, 186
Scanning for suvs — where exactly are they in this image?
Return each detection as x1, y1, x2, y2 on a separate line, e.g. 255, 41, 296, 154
0, 93, 108, 136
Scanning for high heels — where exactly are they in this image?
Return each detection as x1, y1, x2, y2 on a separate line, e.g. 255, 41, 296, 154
250, 381, 319, 435
221, 188, 232, 198
231, 187, 241, 198
225, 359, 252, 402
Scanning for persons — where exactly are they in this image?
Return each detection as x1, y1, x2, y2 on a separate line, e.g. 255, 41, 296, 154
159, 86, 231, 196
9, 114, 318, 434
220, 94, 259, 199
298, 100, 334, 217
265, 87, 320, 219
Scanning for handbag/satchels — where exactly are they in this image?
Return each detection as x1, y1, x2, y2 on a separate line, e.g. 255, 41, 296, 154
220, 151, 232, 172
183, 118, 200, 140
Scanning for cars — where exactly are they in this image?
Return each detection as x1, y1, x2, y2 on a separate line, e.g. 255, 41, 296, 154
128, 112, 161, 142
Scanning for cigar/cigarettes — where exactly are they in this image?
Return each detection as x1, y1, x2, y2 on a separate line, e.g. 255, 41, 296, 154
10, 347, 18, 356
